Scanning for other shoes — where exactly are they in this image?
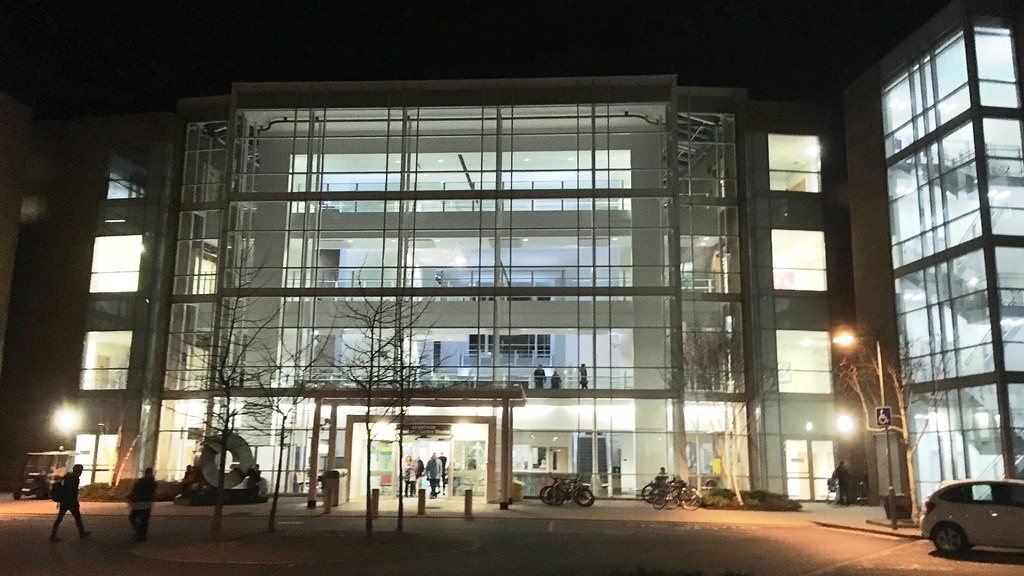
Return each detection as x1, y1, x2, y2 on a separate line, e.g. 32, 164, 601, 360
79, 531, 92, 539
50, 536, 61, 542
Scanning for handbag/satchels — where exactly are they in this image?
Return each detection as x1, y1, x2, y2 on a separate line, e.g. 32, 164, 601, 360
401, 470, 409, 480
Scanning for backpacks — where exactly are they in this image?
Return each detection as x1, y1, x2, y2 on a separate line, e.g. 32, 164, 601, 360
50, 478, 65, 502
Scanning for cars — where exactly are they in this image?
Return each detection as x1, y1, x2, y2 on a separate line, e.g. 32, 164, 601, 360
919, 478, 1024, 558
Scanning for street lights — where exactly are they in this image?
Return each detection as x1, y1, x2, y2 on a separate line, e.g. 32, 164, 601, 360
832, 331, 897, 522
55, 407, 101, 483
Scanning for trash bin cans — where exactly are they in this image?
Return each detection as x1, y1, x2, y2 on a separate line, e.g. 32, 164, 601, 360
884, 494, 912, 520
513, 481, 524, 501
320, 469, 348, 506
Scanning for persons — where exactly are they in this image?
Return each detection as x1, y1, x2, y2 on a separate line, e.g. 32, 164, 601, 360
835, 461, 851, 506
49, 463, 91, 541
401, 451, 446, 499
534, 364, 546, 389
128, 467, 156, 541
580, 364, 588, 389
551, 371, 562, 388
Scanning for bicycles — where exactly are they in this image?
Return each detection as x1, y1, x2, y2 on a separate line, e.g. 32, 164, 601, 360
652, 481, 702, 511
547, 474, 593, 507
642, 480, 698, 506
540, 475, 593, 506
